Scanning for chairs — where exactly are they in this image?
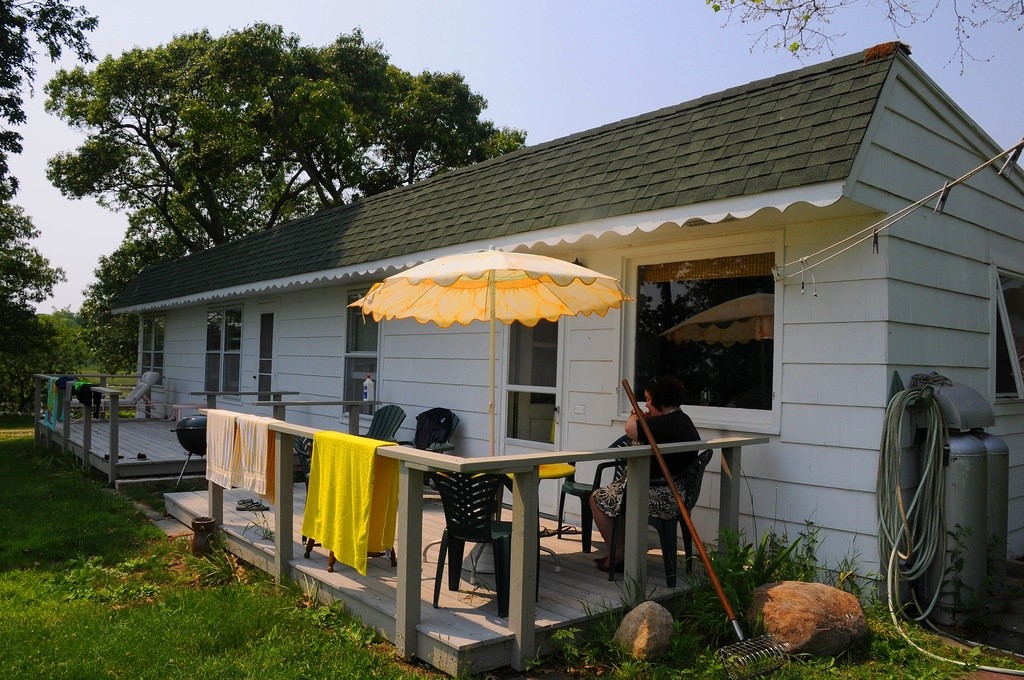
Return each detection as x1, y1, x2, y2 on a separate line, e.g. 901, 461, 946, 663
356, 404, 407, 442
395, 407, 461, 452
70, 371, 175, 424
294, 435, 313, 541
557, 434, 633, 554
607, 449, 713, 589
424, 471, 541, 619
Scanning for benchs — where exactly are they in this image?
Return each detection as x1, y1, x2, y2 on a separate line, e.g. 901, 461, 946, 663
171, 403, 207, 425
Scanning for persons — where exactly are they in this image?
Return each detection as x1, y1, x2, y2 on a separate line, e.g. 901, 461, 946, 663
589, 375, 701, 574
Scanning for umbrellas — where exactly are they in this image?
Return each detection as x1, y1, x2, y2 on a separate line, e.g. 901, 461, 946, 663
345, 244, 637, 456
659, 292, 774, 348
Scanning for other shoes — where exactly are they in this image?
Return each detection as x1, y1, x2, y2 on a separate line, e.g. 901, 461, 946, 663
593, 555, 624, 573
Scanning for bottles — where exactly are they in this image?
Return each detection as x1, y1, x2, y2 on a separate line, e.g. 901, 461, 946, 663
362, 376, 375, 402
702, 387, 710, 406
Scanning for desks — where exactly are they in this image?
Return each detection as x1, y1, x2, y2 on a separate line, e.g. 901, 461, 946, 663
190, 390, 300, 409
421, 463, 576, 583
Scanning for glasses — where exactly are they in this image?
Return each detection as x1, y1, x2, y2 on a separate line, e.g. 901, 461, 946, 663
649, 377, 659, 385
